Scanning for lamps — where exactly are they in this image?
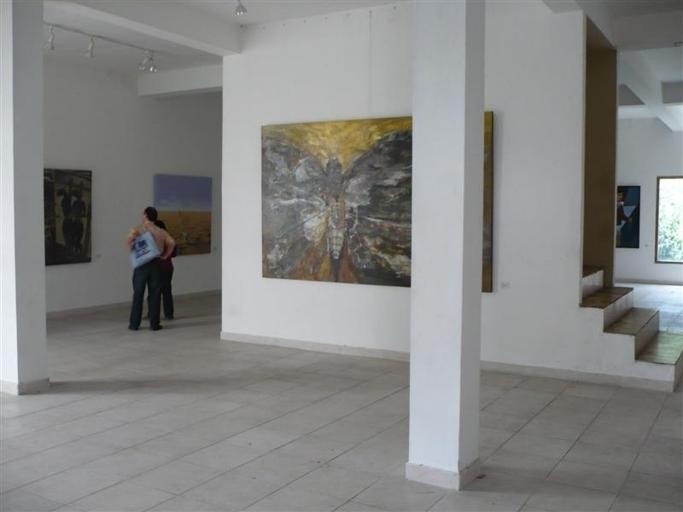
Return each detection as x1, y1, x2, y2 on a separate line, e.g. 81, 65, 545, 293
232, 0, 247, 16
42, 22, 160, 74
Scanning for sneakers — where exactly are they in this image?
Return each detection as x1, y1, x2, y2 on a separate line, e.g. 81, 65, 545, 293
128, 324, 138, 330
152, 325, 162, 331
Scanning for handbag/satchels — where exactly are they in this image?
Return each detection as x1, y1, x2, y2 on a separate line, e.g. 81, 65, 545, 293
129, 231, 160, 268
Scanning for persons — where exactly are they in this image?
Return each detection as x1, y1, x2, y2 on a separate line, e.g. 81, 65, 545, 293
616, 190, 632, 245
143, 220, 174, 320
127, 206, 174, 331
